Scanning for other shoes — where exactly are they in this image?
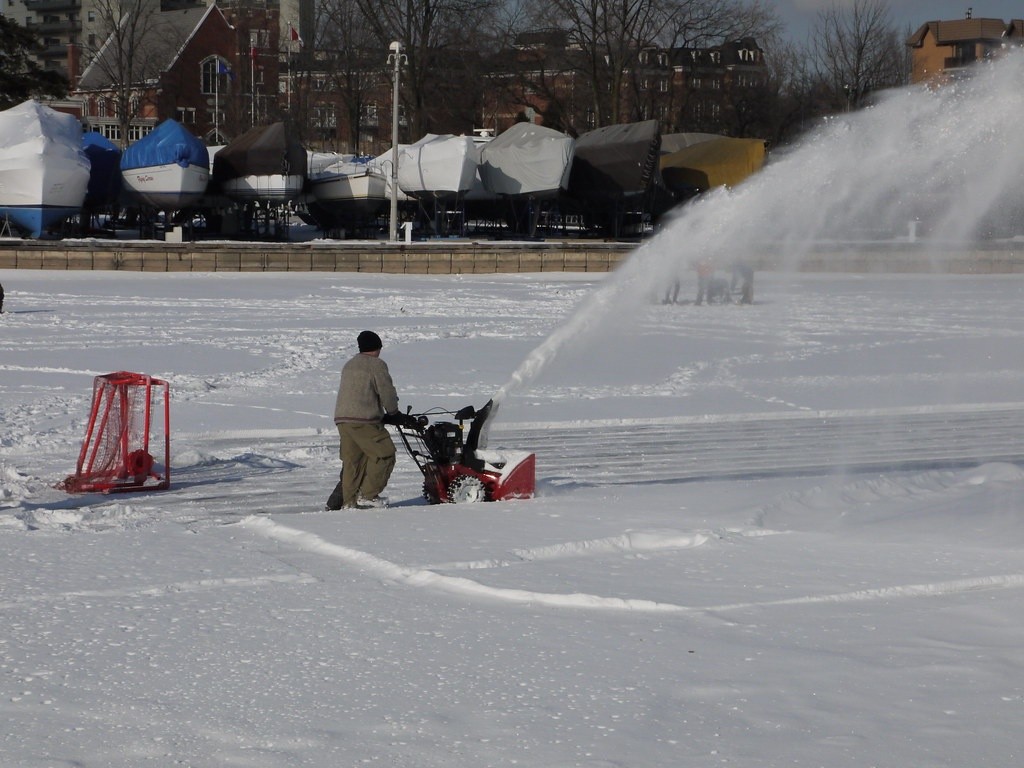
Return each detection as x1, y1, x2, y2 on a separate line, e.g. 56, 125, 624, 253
343, 493, 389, 511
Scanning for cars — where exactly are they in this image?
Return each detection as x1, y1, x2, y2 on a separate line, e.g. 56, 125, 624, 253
352, 154, 376, 163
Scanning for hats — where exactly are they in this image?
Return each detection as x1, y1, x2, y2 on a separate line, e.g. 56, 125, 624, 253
357, 331, 382, 352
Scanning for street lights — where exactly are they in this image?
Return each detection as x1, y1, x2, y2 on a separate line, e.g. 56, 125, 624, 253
387, 41, 408, 241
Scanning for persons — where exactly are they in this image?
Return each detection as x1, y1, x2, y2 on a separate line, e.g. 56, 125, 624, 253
651, 268, 755, 306
334, 330, 404, 508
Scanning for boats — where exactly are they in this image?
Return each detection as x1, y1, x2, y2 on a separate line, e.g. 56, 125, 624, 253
0, 99, 91, 239
573, 119, 662, 201
118, 118, 209, 211
398, 133, 478, 210
81, 131, 122, 209
214, 122, 308, 206
661, 133, 770, 198
476, 122, 575, 200
290, 168, 387, 225
